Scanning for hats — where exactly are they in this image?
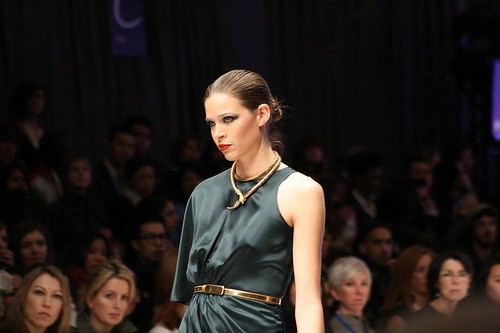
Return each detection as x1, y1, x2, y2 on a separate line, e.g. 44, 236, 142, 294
471, 202, 498, 217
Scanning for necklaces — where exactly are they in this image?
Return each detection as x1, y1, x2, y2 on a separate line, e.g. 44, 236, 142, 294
233, 150, 280, 182
227, 155, 283, 210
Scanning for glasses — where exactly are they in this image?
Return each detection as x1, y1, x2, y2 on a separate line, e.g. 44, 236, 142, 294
138, 232, 170, 241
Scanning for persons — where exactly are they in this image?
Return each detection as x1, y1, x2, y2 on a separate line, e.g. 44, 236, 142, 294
0, 81, 500, 333
170, 69, 326, 333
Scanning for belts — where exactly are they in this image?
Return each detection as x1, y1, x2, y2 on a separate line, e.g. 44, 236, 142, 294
193, 285, 281, 305
